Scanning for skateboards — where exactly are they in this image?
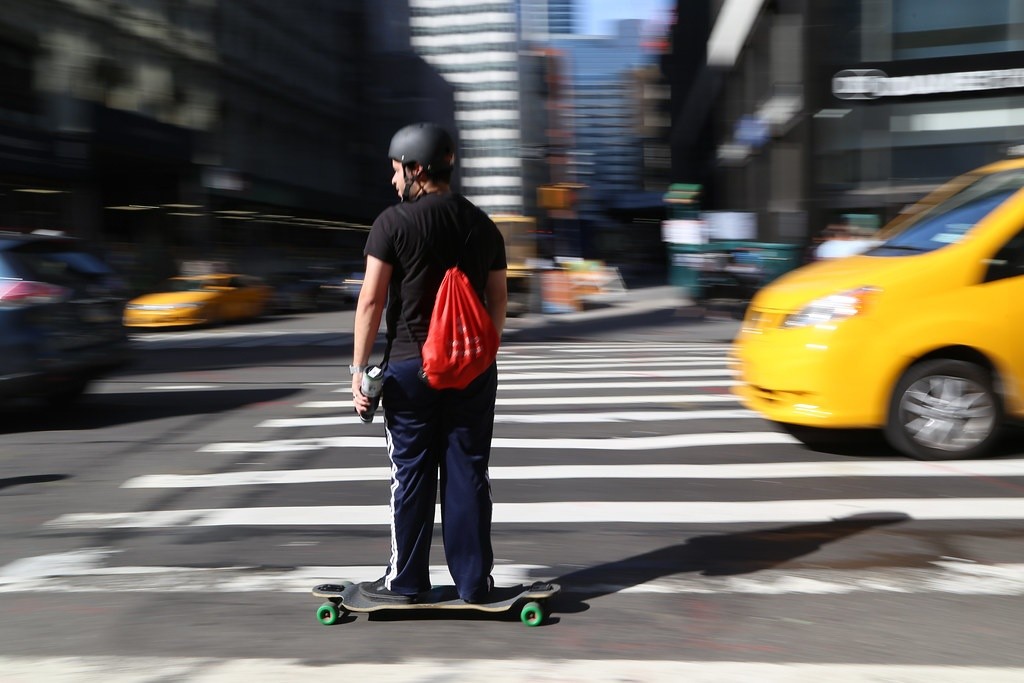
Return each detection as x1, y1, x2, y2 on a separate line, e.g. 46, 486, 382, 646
314, 580, 561, 626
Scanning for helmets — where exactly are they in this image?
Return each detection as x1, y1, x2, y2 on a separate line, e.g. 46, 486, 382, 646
389, 123, 454, 173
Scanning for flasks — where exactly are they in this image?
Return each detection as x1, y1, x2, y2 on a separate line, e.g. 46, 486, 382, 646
359, 367, 384, 425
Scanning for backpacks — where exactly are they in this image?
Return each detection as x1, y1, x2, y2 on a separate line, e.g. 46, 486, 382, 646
393, 198, 497, 390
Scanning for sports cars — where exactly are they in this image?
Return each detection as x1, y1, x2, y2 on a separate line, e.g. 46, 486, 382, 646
120, 272, 273, 330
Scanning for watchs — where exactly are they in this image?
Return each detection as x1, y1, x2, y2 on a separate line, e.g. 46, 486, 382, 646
349, 364, 366, 375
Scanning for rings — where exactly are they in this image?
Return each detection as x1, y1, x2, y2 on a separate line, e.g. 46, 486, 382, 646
353, 393, 357, 399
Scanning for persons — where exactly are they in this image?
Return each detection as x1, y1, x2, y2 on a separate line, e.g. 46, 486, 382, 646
348, 120, 510, 604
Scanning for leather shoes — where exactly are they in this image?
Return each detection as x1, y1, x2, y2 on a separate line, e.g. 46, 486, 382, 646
359, 577, 417, 605
464, 591, 494, 604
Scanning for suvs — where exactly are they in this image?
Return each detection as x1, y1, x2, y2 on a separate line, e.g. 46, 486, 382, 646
726, 144, 1023, 461
0, 230, 129, 424
